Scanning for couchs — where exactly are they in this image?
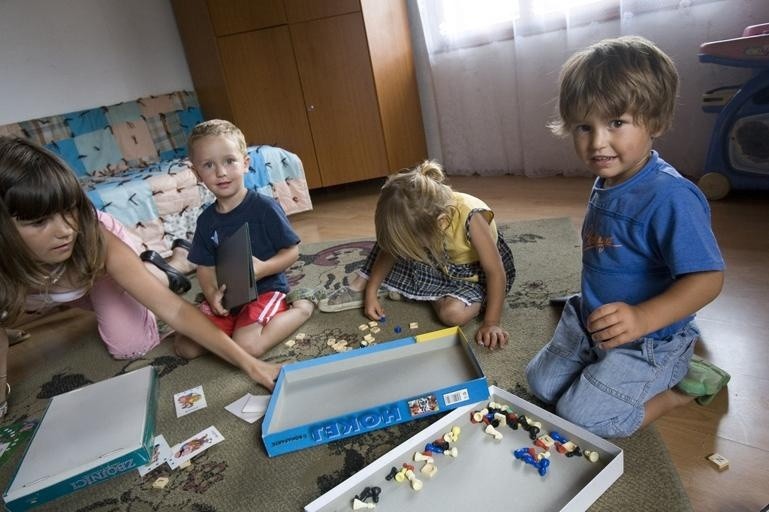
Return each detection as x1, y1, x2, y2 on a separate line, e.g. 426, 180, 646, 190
1, 88, 299, 258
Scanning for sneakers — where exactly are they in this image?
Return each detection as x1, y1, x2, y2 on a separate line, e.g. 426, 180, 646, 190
676, 353, 731, 406
285, 286, 366, 312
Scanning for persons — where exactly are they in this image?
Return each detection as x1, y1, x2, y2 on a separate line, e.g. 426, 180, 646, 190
317, 157, 516, 351
523, 34, 731, 439
174, 118, 327, 358
0, 131, 283, 422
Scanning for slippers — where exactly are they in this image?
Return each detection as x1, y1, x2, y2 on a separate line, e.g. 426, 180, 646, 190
3, 328, 30, 346
171, 238, 192, 252
140, 250, 191, 292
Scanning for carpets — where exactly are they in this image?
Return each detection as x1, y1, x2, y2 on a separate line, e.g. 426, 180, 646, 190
0, 214, 698, 511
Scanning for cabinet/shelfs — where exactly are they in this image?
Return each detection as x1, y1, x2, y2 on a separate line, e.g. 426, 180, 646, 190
170, 2, 427, 192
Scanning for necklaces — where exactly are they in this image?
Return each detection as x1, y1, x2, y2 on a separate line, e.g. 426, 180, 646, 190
41, 261, 68, 283
